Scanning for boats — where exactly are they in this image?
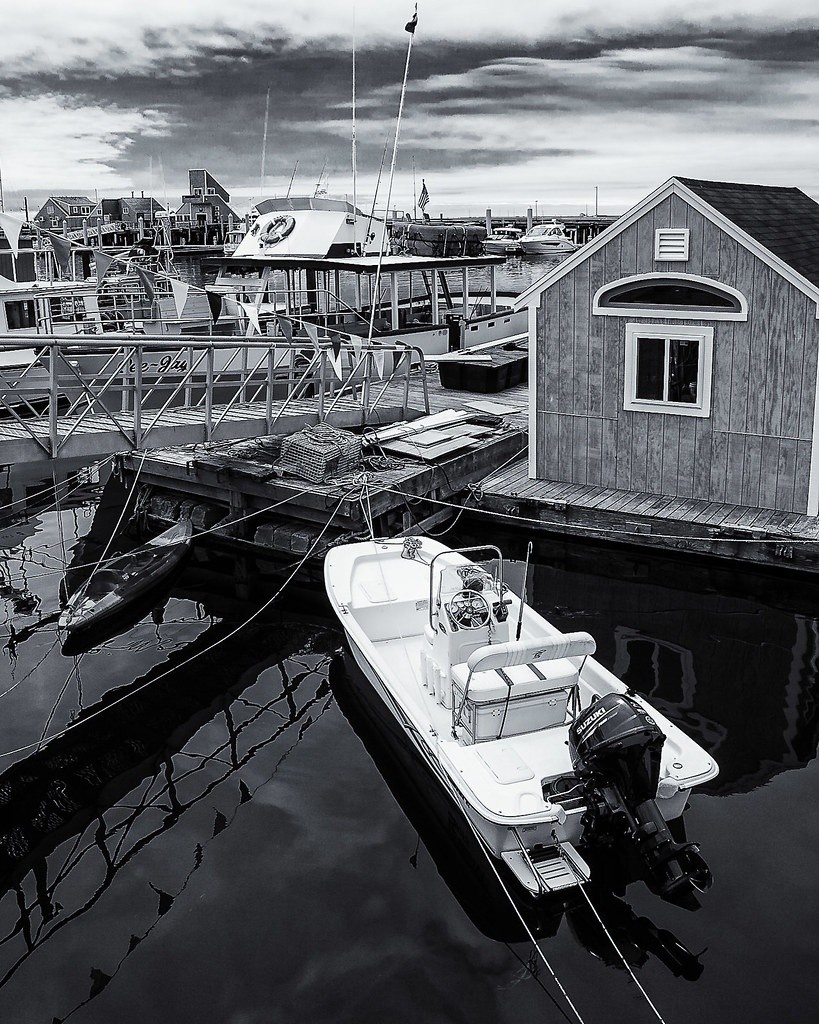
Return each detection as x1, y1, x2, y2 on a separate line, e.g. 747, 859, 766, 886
0, 271, 218, 414
518, 225, 577, 256
58, 518, 194, 630
321, 535, 725, 909
33, 195, 530, 420
481, 225, 525, 253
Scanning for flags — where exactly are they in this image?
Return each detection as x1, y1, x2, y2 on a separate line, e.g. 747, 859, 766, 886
405, 13, 418, 34
417, 184, 429, 210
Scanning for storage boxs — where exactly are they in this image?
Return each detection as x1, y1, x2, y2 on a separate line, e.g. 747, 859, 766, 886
464, 691, 569, 740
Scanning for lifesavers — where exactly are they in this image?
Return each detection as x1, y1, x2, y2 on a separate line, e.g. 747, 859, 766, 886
260, 215, 296, 243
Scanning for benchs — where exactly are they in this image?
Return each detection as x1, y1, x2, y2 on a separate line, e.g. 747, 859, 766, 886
451, 631, 596, 741
157, 294, 236, 334
